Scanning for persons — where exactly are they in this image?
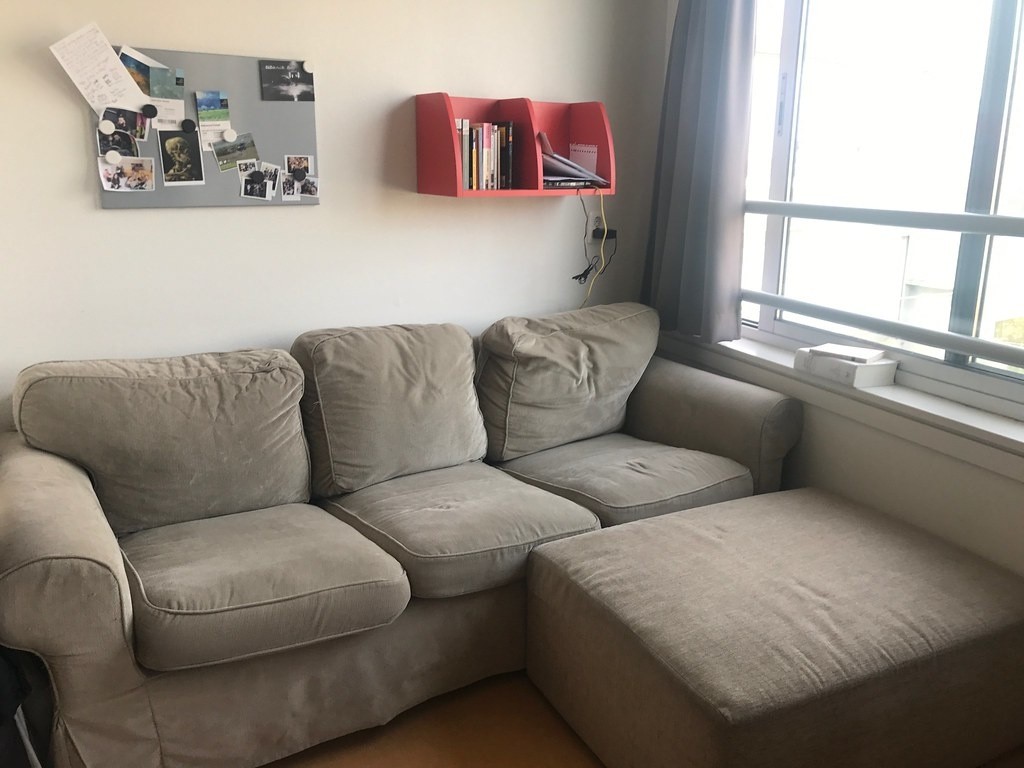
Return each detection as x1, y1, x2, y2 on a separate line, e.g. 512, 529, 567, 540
101, 135, 134, 156
247, 183, 260, 196
288, 157, 308, 173
103, 169, 112, 182
300, 179, 317, 194
283, 178, 294, 195
236, 139, 246, 151
125, 169, 153, 190
240, 162, 255, 177
110, 174, 121, 189
116, 166, 125, 178
263, 167, 279, 190
113, 107, 138, 136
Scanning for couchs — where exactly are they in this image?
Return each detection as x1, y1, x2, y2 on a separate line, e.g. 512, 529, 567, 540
0, 357, 804, 768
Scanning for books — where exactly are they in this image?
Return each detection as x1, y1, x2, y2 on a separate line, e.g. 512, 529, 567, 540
453, 118, 513, 191
793, 342, 899, 388
540, 131, 609, 188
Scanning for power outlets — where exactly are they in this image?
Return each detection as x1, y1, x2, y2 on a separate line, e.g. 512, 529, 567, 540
586, 210, 606, 245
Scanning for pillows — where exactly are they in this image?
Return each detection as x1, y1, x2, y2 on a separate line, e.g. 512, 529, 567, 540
290, 322, 488, 498
12, 348, 312, 537
475, 302, 661, 463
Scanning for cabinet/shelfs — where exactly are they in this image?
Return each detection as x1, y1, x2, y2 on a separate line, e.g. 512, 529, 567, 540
413, 93, 618, 196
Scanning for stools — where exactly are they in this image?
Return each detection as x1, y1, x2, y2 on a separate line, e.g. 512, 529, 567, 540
525, 485, 1024, 768
